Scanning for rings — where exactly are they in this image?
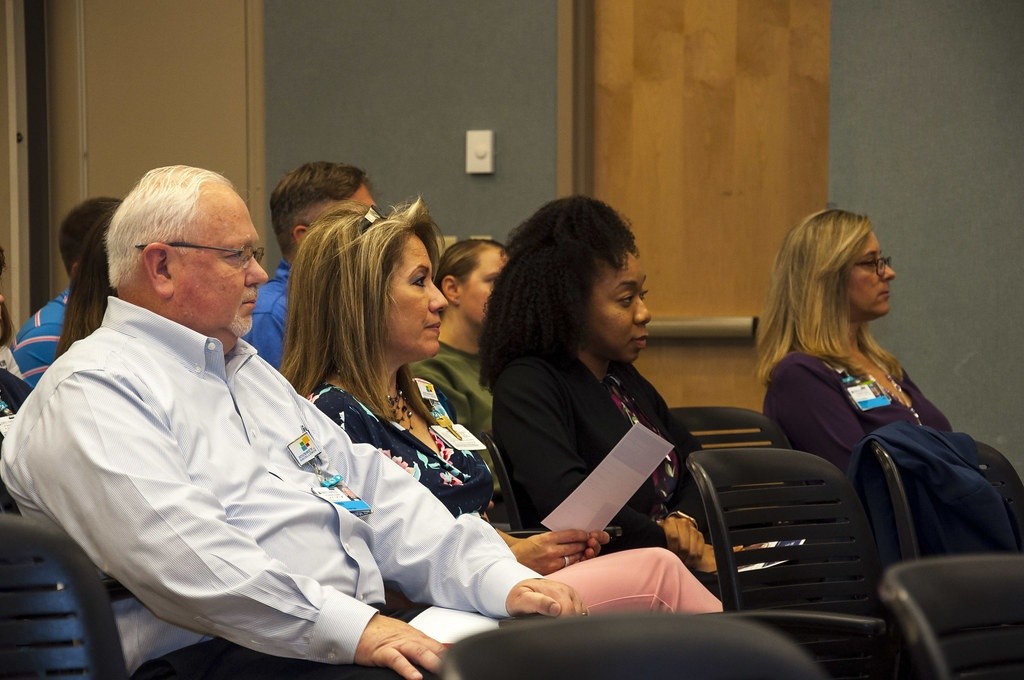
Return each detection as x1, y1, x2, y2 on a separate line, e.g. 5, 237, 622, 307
582, 612, 587, 616
564, 556, 569, 567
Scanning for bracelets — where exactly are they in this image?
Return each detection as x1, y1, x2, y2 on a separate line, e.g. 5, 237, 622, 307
666, 511, 698, 531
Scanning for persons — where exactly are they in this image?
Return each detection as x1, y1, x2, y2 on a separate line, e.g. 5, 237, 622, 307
0, 161, 511, 539
477, 195, 745, 613
279, 195, 723, 619
1, 165, 589, 680
756, 208, 953, 522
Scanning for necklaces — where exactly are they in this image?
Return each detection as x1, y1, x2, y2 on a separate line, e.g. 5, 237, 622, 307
385, 390, 414, 432
868, 373, 922, 426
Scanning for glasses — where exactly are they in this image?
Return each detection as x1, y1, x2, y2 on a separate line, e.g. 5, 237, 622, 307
361, 204, 389, 230
135, 242, 265, 265
853, 256, 893, 278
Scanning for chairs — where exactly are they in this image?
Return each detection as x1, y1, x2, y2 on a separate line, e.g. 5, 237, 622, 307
0, 509, 128, 680
436, 406, 1024, 680
